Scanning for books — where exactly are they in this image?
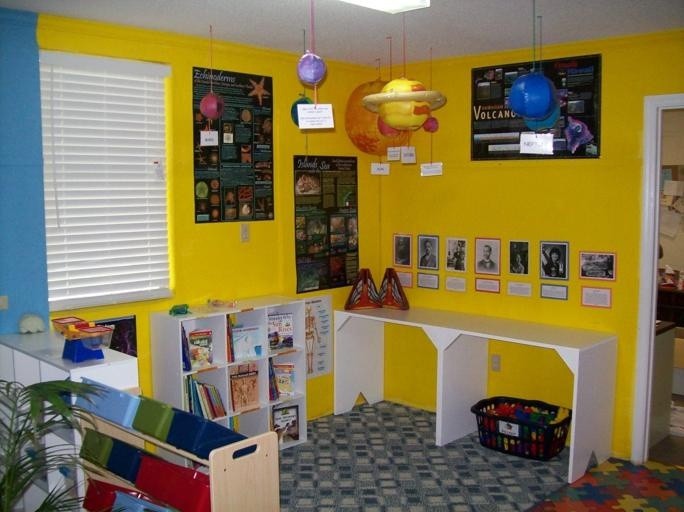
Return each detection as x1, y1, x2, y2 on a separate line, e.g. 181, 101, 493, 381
179, 314, 301, 448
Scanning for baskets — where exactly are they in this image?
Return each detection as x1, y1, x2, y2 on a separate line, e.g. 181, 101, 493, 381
470, 394, 573, 462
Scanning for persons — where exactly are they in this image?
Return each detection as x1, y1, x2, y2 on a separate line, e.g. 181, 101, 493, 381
419, 240, 435, 268
395, 238, 409, 265
476, 244, 495, 271
543, 247, 563, 277
510, 251, 525, 274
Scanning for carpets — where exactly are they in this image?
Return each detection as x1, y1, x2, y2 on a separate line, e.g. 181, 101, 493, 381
528, 459, 684, 511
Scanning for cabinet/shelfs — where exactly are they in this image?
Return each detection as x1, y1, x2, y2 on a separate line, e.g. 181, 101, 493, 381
78, 409, 282, 512
151, 294, 308, 451
0, 332, 139, 512
649, 327, 676, 450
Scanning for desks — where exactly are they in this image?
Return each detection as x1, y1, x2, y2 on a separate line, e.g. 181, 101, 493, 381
331, 308, 618, 484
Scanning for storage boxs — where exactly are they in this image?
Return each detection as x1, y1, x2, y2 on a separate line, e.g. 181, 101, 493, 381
74, 375, 255, 512
